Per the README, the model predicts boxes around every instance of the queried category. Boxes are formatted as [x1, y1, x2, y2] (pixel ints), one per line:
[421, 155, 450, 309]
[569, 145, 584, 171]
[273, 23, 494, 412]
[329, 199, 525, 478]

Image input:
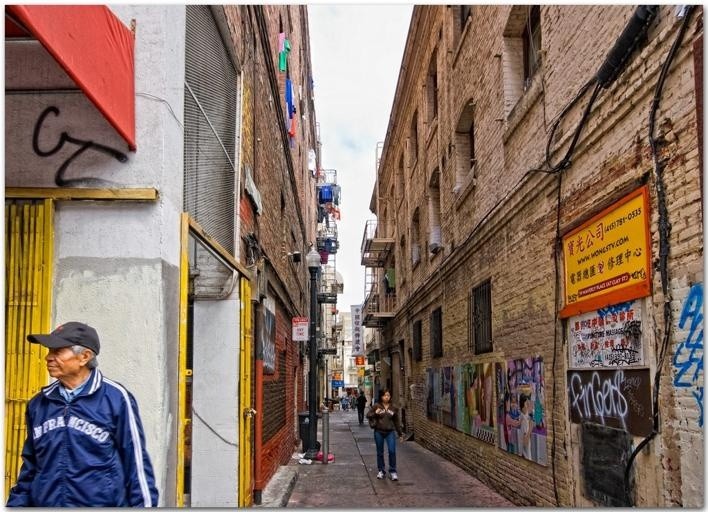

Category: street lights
[305, 245, 322, 461]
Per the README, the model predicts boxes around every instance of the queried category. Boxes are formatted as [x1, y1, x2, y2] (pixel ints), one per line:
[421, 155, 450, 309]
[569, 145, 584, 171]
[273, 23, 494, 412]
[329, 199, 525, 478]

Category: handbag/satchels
[369, 418, 377, 428]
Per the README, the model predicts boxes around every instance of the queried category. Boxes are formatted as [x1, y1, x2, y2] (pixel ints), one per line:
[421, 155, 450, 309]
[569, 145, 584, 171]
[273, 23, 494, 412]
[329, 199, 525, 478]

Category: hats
[27, 321, 100, 355]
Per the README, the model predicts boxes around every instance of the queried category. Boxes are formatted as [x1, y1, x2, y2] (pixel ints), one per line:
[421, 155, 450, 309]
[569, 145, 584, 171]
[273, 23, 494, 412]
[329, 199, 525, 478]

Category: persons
[467, 372, 486, 433]
[366, 388, 403, 480]
[5, 321, 158, 507]
[504, 371, 543, 460]
[356, 390, 368, 424]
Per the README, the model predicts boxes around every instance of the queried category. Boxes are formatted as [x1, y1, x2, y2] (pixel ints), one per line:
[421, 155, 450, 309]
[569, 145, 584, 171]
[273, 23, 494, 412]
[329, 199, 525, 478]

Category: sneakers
[377, 470, 398, 481]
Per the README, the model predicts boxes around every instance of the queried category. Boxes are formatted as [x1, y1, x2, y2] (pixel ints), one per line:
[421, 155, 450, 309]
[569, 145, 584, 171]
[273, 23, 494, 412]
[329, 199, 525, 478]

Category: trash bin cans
[298, 411, 321, 453]
